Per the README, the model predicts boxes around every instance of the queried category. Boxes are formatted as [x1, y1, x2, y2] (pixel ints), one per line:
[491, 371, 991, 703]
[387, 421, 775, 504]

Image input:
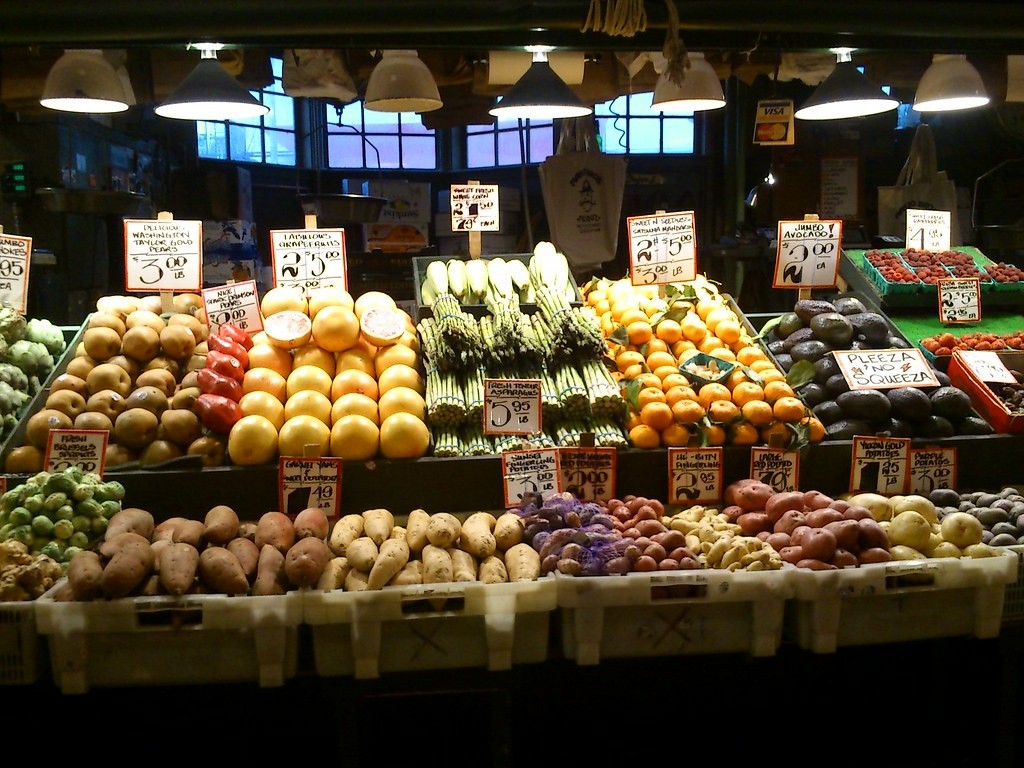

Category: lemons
[228, 286, 430, 460]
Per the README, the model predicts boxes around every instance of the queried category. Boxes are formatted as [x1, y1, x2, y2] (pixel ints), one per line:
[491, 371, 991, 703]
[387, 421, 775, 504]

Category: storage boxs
[947, 267, 994, 306]
[911, 267, 955, 308]
[0, 578, 65, 687]
[742, 312, 996, 494]
[412, 253, 584, 325]
[933, 251, 978, 269]
[948, 349, 1024, 434]
[918, 338, 969, 371]
[304, 513, 556, 679]
[555, 562, 800, 666]
[793, 547, 1018, 653]
[897, 252, 943, 269]
[34, 576, 304, 696]
[861, 251, 906, 282]
[981, 263, 1024, 304]
[874, 268, 922, 309]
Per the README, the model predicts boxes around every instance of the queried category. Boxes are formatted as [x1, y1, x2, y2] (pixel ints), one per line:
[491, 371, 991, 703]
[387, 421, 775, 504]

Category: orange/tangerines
[575, 272, 825, 447]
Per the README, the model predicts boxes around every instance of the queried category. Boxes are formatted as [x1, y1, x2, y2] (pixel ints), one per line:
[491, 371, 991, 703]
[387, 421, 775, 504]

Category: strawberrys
[920, 330, 1024, 356]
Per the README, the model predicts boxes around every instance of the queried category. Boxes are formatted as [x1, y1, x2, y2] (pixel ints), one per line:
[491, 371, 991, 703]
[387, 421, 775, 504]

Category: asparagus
[416, 287, 630, 457]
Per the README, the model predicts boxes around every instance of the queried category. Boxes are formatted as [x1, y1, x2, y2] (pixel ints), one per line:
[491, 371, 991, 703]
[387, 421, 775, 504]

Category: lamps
[364, 49, 443, 113]
[649, 53, 726, 111]
[39, 47, 129, 113]
[795, 47, 901, 121]
[154, 49, 270, 120]
[487, 47, 593, 119]
[913, 53, 989, 111]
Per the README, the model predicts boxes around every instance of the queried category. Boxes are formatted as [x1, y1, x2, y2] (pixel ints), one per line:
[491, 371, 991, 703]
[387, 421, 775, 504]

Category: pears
[6, 293, 253, 466]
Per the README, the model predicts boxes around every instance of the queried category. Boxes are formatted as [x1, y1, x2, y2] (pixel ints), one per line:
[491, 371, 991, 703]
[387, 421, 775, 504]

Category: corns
[422, 242, 575, 308]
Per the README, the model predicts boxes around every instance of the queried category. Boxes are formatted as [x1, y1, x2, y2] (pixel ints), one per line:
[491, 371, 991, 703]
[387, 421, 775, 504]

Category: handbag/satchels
[878, 123, 963, 247]
[538, 115, 626, 274]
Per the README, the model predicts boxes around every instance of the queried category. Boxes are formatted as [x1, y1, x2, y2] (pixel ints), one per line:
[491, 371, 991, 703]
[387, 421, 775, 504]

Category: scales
[971, 159, 1024, 250]
[293, 48, 388, 226]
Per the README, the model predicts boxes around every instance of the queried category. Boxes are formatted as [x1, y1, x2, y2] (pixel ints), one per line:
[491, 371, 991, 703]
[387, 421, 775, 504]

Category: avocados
[764, 297, 994, 442]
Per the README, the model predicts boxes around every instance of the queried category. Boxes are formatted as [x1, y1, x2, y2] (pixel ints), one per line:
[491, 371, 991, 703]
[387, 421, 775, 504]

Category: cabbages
[0, 466, 127, 574]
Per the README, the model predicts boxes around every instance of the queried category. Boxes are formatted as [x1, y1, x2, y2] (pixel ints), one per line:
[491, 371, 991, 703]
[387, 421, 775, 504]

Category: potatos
[67, 508, 541, 634]
[528, 478, 1024, 598]
